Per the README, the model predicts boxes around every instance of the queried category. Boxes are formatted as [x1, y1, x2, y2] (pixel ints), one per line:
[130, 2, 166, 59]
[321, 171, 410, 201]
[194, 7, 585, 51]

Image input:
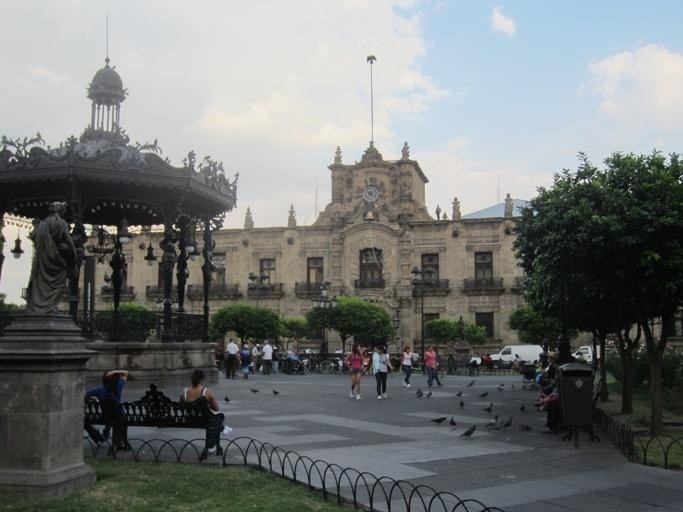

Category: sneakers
[541, 426, 556, 433]
[350, 390, 387, 400]
[207, 445, 217, 453]
[223, 427, 234, 435]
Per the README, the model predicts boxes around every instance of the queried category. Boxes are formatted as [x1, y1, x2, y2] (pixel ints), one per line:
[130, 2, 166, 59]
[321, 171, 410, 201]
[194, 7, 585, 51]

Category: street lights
[248, 270, 269, 308]
[318, 280, 332, 339]
[410, 266, 434, 373]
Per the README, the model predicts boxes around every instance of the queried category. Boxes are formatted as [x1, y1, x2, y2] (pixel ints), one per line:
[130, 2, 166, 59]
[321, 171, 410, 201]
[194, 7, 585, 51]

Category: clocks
[363, 185, 379, 202]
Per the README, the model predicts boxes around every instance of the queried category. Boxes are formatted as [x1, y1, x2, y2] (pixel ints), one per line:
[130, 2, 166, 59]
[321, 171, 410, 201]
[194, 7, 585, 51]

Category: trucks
[571, 346, 603, 363]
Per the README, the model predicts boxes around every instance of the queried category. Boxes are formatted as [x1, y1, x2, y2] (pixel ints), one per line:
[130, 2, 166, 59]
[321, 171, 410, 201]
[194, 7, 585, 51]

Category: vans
[489, 344, 545, 366]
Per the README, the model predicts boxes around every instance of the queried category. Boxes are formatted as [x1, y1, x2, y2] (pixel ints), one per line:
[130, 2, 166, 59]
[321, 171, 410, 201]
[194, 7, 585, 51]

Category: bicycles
[304, 350, 335, 374]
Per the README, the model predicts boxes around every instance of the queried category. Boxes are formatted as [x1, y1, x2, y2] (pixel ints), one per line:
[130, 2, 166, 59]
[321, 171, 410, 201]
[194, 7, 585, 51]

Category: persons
[369, 345, 394, 400]
[343, 342, 363, 400]
[400, 346, 413, 388]
[521, 349, 565, 435]
[224, 338, 522, 383]
[25, 201, 79, 314]
[422, 345, 442, 389]
[178, 370, 233, 454]
[102, 368, 132, 453]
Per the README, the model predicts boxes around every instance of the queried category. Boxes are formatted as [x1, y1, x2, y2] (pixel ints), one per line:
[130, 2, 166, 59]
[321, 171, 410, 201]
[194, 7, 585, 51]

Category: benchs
[85, 382, 225, 461]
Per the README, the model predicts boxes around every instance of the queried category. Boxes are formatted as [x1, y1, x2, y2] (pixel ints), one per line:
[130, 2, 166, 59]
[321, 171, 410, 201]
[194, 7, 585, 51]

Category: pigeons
[416, 383, 531, 438]
[272, 389, 280, 396]
[224, 396, 230, 402]
[250, 388, 259, 394]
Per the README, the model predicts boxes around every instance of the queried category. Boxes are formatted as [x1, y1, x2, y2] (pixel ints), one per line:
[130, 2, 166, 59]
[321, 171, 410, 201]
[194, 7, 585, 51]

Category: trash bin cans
[556, 362, 594, 430]
